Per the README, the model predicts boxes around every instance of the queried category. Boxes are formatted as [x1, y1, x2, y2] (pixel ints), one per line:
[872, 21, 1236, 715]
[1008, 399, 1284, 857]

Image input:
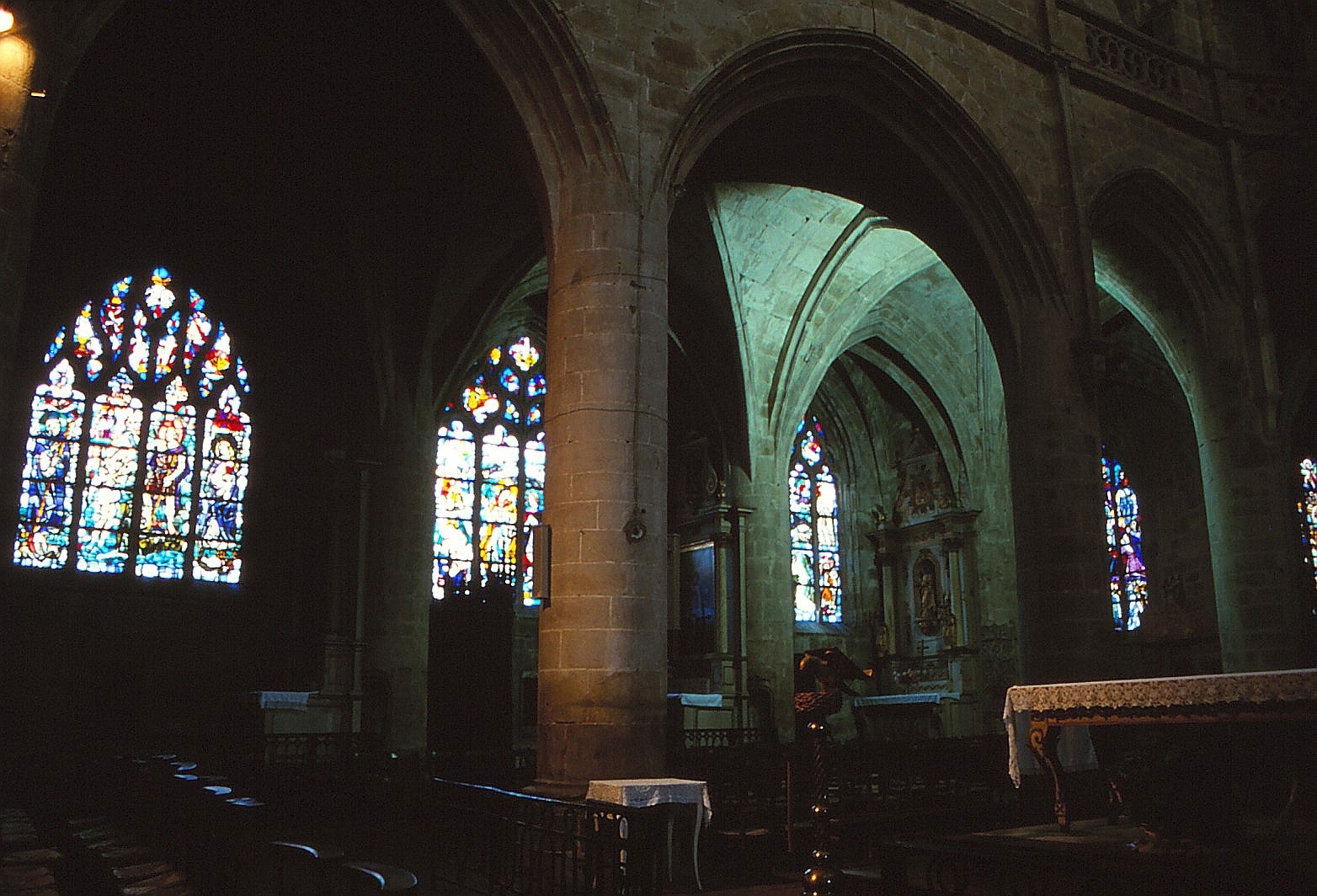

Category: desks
[585, 778, 713, 894]
[847, 692, 961, 740]
[1002, 668, 1316, 829]
